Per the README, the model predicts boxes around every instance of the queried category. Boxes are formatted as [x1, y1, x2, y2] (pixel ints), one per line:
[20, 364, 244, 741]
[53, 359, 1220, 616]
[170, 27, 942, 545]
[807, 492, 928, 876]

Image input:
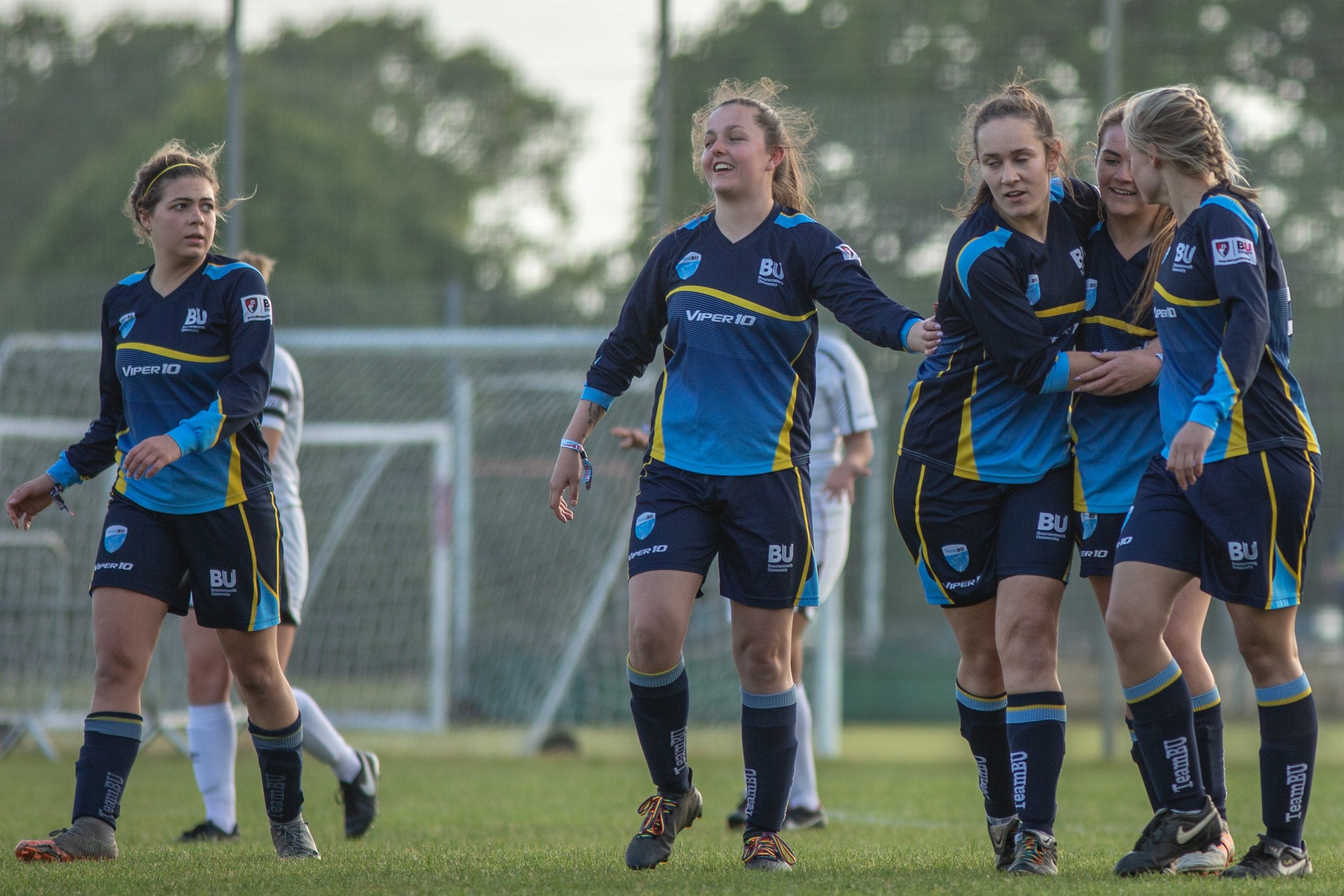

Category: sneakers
[988, 818, 1023, 872]
[1218, 833, 1313, 880]
[1006, 829, 1058, 877]
[1115, 794, 1223, 878]
[625, 784, 703, 870]
[178, 818, 240, 843]
[334, 750, 380, 841]
[15, 827, 118, 865]
[1176, 818, 1236, 876]
[741, 829, 796, 872]
[270, 821, 321, 861]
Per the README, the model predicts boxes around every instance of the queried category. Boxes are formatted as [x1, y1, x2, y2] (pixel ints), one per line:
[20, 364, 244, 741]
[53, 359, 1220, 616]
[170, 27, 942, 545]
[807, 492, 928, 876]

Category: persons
[5, 151, 319, 862]
[179, 249, 379, 843]
[549, 67, 1320, 884]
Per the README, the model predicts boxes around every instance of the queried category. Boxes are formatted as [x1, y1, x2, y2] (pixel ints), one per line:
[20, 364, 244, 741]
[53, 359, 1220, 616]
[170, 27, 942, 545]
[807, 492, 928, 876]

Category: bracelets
[46, 470, 74, 516]
[560, 439, 593, 490]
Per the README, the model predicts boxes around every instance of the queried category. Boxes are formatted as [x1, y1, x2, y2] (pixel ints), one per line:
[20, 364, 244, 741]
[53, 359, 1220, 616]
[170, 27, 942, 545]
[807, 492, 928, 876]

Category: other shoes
[728, 796, 750, 831]
[783, 802, 829, 832]
